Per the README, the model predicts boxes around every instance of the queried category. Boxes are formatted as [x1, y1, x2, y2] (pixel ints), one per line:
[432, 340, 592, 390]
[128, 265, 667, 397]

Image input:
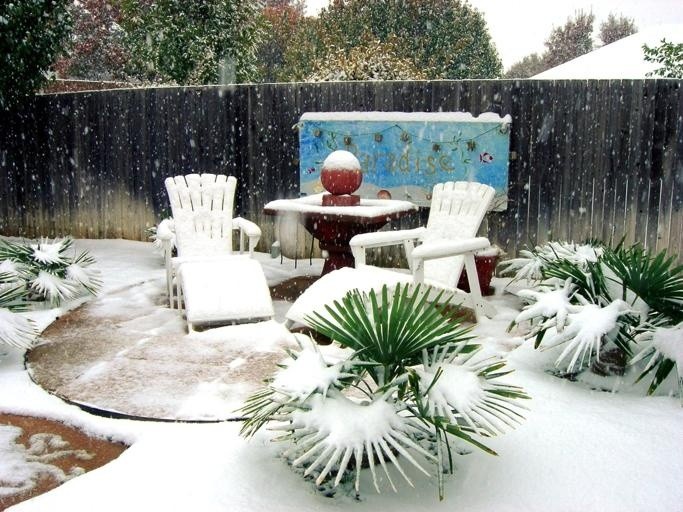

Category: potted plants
[495, 231, 683, 400]
